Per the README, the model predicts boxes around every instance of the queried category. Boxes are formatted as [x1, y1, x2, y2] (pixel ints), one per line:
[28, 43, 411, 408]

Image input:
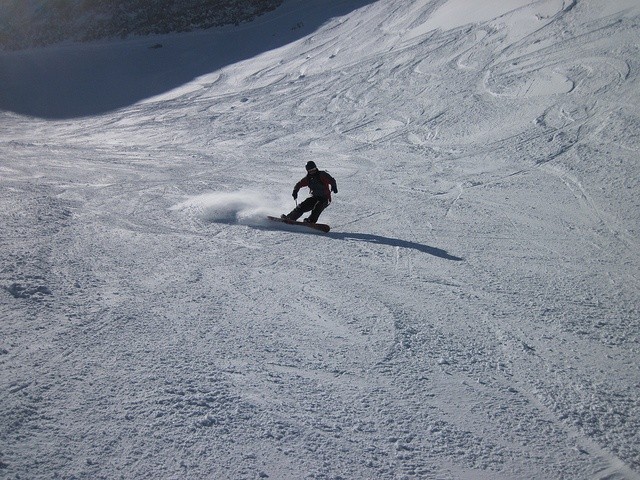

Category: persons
[281, 160, 338, 224]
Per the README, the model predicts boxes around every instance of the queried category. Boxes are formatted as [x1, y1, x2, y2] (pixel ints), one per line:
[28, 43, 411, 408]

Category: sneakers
[304, 218, 315, 224]
[281, 214, 296, 223]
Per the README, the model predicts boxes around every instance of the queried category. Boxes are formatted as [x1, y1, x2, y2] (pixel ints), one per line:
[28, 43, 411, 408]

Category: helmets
[305, 161, 317, 173]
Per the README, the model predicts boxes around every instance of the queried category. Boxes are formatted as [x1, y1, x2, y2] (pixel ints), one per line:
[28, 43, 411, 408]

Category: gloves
[330, 187, 338, 194]
[292, 191, 298, 200]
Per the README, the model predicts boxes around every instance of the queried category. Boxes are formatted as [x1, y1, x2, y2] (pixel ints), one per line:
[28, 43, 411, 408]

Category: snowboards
[267, 216, 330, 233]
